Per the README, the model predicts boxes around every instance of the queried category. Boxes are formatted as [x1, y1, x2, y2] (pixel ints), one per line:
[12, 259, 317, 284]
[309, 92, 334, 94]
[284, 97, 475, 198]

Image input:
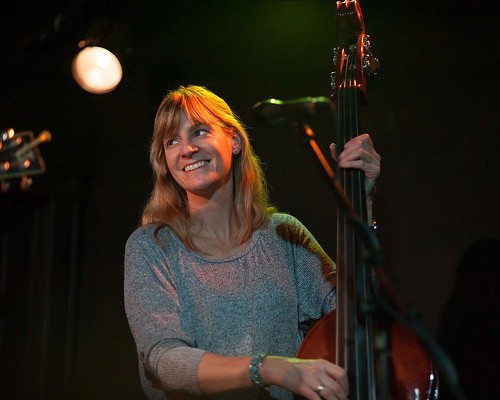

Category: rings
[317, 385, 325, 393]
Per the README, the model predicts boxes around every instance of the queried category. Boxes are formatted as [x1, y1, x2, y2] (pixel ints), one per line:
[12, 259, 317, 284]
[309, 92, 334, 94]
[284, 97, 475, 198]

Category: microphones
[252, 97, 337, 129]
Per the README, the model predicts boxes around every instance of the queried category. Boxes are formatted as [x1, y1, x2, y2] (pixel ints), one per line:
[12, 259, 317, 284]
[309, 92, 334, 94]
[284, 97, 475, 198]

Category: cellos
[284, 1, 443, 400]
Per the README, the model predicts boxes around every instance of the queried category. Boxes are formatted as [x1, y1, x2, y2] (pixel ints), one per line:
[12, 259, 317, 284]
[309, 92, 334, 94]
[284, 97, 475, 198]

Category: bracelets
[249, 353, 269, 389]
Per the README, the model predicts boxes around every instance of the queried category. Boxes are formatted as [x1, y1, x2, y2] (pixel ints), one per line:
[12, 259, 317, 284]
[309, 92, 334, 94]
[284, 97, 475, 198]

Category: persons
[122, 84, 380, 400]
